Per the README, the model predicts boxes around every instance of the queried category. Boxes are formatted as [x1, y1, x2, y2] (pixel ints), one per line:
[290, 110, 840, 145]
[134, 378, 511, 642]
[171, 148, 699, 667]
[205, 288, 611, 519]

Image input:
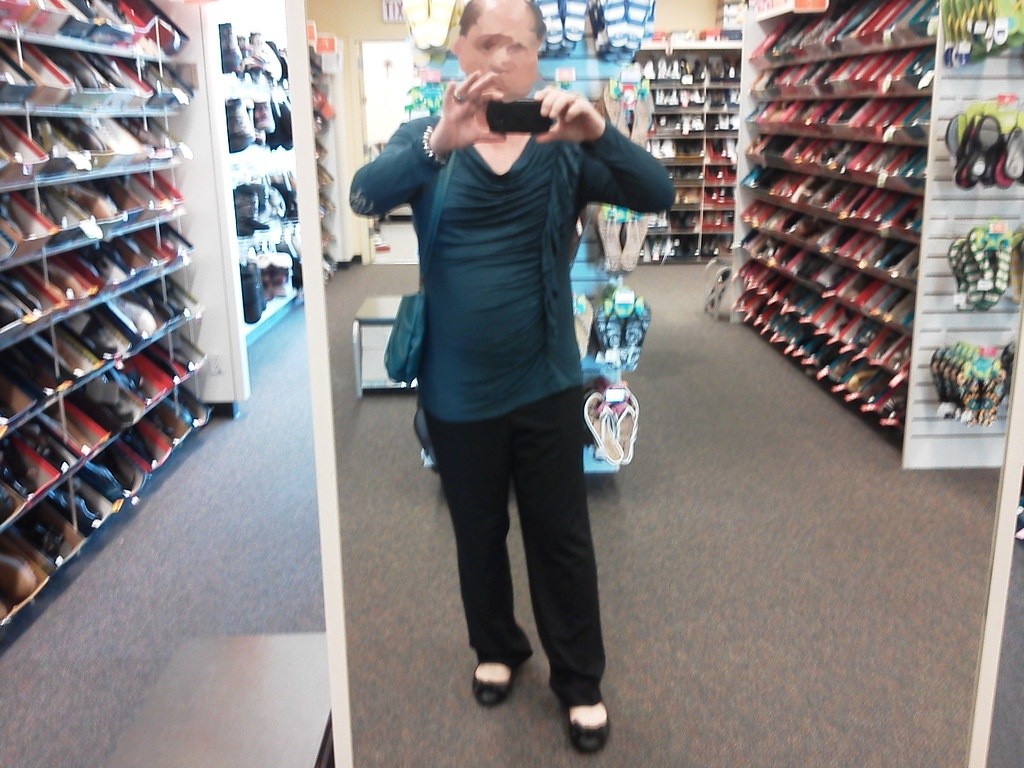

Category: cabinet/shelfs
[306, 0, 1024, 768]
[644, 0, 1022, 470]
[0, 0, 300, 654]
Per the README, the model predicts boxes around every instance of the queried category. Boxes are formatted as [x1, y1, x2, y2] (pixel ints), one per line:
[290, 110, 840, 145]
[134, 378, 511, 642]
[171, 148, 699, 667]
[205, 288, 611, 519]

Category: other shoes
[567, 702, 611, 754]
[472, 662, 518, 708]
[217, 23, 303, 324]
[620, 31, 741, 263]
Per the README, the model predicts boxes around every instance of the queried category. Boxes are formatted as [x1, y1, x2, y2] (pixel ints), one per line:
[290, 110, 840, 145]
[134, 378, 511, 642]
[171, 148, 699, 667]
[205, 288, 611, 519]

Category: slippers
[572, 284, 652, 372]
[945, 100, 1024, 191]
[930, 340, 1016, 429]
[597, 203, 649, 273]
[600, 77, 655, 151]
[582, 383, 640, 466]
[948, 220, 1024, 313]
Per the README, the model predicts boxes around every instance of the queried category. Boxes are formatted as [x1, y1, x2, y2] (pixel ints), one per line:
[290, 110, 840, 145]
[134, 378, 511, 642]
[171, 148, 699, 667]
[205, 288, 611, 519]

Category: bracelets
[424, 125, 449, 165]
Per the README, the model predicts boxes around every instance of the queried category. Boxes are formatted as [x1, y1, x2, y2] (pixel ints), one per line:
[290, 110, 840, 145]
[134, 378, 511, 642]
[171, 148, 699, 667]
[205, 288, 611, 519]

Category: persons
[348, 0, 676, 753]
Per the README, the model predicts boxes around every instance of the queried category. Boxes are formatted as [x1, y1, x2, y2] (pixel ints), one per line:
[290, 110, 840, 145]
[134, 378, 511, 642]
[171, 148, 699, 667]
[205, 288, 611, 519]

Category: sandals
[534, 0, 656, 65]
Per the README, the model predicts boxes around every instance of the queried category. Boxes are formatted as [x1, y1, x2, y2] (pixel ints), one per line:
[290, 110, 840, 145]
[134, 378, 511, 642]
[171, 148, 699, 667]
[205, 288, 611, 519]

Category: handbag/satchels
[383, 291, 425, 389]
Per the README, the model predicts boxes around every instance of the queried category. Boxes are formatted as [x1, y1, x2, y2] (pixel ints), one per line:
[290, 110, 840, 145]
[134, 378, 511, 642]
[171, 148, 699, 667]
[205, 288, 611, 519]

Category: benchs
[352, 295, 419, 398]
[104, 632, 336, 768]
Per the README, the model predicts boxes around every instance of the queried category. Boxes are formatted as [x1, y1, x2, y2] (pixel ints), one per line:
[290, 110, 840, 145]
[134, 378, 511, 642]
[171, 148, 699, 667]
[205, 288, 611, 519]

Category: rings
[452, 92, 466, 103]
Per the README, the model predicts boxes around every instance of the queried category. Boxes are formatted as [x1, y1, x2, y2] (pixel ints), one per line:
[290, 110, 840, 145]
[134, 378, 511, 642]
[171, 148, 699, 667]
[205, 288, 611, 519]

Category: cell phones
[488, 98, 555, 133]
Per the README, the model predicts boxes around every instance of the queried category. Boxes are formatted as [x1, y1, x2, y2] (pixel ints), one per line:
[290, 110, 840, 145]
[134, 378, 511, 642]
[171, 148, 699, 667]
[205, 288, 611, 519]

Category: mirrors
[284, 0, 1024, 768]
